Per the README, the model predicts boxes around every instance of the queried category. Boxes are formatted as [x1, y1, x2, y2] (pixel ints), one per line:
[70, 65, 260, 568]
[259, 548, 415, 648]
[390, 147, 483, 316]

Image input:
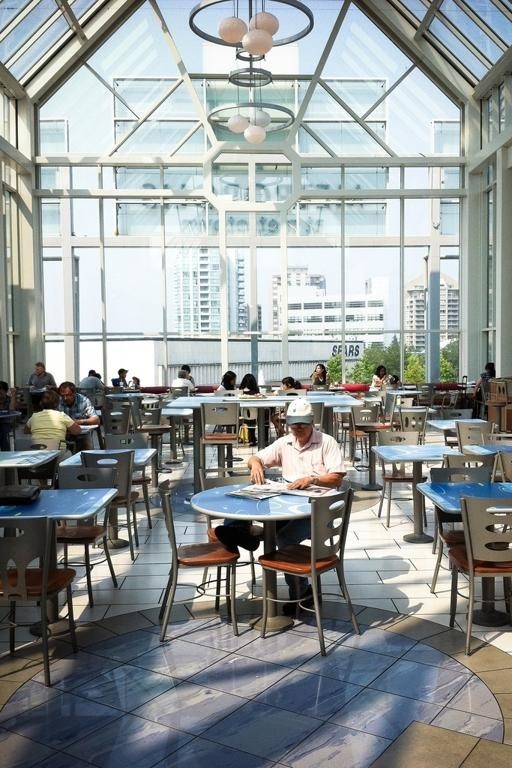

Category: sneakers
[249, 440, 257, 446]
[215, 524, 260, 551]
[282, 585, 314, 613]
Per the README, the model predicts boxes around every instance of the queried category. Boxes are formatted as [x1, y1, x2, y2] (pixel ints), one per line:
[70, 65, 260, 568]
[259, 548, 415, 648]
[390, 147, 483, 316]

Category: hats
[286, 398, 313, 424]
[118, 368, 128, 374]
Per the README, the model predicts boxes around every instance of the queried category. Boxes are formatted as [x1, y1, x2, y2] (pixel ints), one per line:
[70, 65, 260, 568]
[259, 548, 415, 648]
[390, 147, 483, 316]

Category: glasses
[290, 423, 307, 429]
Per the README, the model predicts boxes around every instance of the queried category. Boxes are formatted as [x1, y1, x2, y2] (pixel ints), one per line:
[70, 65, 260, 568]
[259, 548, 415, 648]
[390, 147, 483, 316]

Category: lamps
[207, 46, 295, 143]
[188, 0, 315, 55]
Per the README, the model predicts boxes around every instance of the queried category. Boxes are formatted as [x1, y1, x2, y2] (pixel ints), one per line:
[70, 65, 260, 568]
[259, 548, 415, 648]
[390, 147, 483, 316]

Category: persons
[389, 375, 402, 387]
[471, 362, 496, 420]
[311, 363, 327, 385]
[130, 375, 140, 390]
[171, 370, 194, 396]
[238, 373, 259, 446]
[271, 377, 302, 439]
[215, 371, 237, 396]
[93, 373, 108, 387]
[54, 382, 101, 451]
[214, 398, 345, 617]
[79, 368, 111, 397]
[26, 360, 59, 411]
[117, 367, 129, 388]
[371, 365, 395, 394]
[181, 365, 194, 387]
[0, 380, 18, 452]
[24, 390, 82, 489]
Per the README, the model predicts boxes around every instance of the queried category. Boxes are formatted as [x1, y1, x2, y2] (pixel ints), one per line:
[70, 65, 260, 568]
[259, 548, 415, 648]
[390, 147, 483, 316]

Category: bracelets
[83, 419, 87, 424]
[11, 396, 16, 400]
[311, 474, 320, 486]
